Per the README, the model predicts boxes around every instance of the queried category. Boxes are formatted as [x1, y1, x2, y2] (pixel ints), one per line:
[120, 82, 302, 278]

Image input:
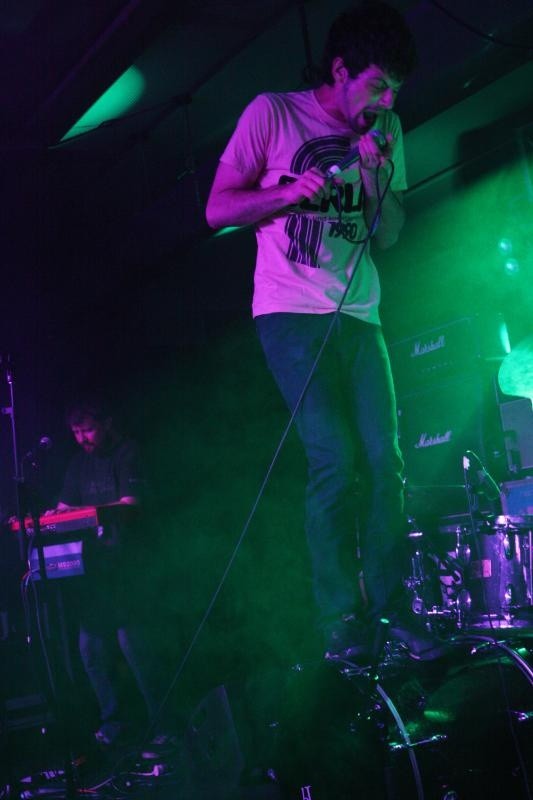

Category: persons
[205, 32, 444, 665]
[42, 402, 170, 791]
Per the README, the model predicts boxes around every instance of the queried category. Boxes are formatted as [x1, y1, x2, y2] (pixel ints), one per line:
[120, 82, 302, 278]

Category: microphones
[467, 450, 501, 501]
[325, 130, 388, 179]
[25, 437, 51, 460]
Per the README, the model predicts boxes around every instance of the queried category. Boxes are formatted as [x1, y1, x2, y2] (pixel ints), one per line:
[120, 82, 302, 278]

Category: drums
[459, 513, 532, 629]
[258, 635, 533, 799]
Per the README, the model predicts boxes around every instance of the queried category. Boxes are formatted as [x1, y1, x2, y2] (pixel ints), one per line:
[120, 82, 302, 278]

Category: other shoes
[94, 716, 122, 743]
[324, 623, 382, 660]
[371, 615, 438, 661]
[140, 729, 177, 759]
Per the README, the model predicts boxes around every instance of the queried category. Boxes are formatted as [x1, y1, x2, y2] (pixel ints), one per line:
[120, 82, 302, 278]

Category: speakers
[392, 318, 533, 518]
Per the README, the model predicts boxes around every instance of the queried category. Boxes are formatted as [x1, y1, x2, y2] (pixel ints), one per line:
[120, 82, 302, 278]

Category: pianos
[10, 501, 134, 717]
